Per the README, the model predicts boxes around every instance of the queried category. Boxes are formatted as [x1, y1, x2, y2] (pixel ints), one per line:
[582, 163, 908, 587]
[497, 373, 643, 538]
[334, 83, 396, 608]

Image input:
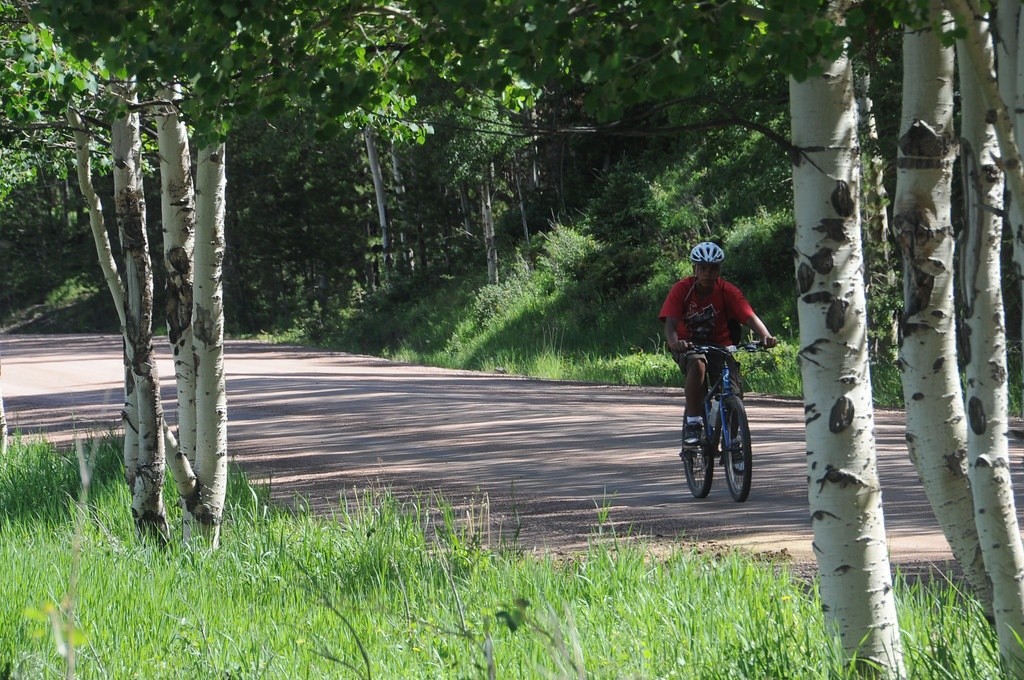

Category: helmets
[691, 241, 725, 265]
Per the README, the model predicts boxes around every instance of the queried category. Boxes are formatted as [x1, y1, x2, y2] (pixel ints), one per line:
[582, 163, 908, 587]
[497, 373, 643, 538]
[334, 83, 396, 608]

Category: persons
[657, 242, 780, 473]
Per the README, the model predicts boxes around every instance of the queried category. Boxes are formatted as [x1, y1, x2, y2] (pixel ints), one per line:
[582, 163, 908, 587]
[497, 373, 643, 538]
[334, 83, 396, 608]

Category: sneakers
[727, 435, 745, 475]
[683, 420, 705, 452]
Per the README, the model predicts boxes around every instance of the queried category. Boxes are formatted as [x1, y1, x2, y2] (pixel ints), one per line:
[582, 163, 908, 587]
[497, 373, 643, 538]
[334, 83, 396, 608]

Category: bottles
[709, 395, 721, 427]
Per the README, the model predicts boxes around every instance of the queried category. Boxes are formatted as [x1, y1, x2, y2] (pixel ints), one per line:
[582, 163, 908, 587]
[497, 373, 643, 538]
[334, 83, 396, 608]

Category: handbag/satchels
[729, 319, 742, 346]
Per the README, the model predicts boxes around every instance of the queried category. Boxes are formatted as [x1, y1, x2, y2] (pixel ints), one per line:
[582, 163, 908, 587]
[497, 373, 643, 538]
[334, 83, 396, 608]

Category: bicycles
[675, 342, 771, 503]
